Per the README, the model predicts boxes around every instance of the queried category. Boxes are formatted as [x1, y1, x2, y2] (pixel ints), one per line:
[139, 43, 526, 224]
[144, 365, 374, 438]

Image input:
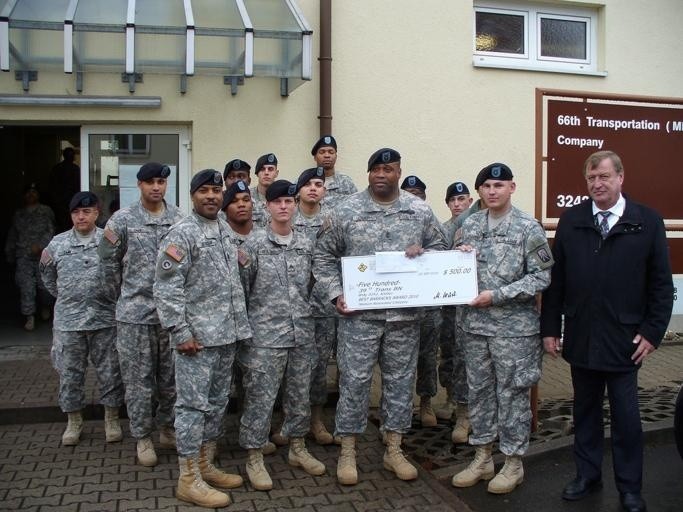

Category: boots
[137, 423, 178, 467]
[25, 309, 50, 330]
[420, 397, 437, 427]
[452, 443, 495, 488]
[104, 404, 123, 442]
[337, 435, 357, 485]
[452, 404, 472, 442]
[198, 444, 243, 488]
[436, 387, 456, 419]
[62, 410, 84, 445]
[383, 432, 418, 480]
[245, 405, 333, 490]
[176, 454, 230, 507]
[488, 455, 524, 493]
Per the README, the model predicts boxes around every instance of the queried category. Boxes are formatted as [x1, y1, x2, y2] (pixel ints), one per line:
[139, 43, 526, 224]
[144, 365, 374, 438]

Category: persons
[3, 180, 64, 332]
[47, 148, 80, 232]
[539, 150, 673, 512]
[96, 162, 189, 466]
[451, 163, 554, 495]
[152, 168, 244, 509]
[38, 193, 123, 446]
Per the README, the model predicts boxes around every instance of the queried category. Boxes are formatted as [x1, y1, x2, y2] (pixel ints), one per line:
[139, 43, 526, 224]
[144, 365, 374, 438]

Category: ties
[599, 213, 613, 239]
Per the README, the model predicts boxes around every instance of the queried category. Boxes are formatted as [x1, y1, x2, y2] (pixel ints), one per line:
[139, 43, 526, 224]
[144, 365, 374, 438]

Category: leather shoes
[620, 492, 646, 512]
[563, 477, 603, 499]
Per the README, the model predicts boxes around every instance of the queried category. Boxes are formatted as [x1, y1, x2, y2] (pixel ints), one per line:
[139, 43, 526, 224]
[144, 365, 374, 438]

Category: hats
[368, 148, 400, 172]
[297, 167, 325, 190]
[265, 180, 296, 201]
[224, 159, 250, 180]
[401, 176, 426, 190]
[475, 163, 512, 190]
[70, 191, 98, 211]
[221, 181, 250, 211]
[190, 169, 223, 193]
[446, 182, 470, 204]
[312, 136, 337, 156]
[137, 162, 170, 180]
[255, 153, 277, 174]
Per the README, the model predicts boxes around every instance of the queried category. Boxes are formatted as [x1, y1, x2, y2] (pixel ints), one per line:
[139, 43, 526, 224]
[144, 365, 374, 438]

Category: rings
[180, 351, 188, 358]
